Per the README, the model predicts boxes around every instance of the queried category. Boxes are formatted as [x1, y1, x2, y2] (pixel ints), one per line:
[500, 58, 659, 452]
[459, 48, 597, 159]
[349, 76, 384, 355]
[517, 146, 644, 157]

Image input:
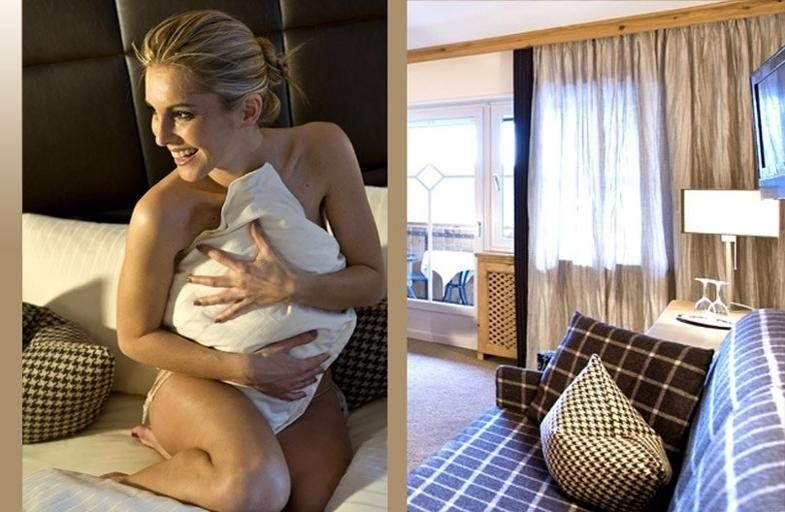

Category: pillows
[22, 302, 116, 443]
[539, 353, 674, 512]
[525, 309, 715, 456]
[327, 300, 388, 414]
[163, 161, 358, 437]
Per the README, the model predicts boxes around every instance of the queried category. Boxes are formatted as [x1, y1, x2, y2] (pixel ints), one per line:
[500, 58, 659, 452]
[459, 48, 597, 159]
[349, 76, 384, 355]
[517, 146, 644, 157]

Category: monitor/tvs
[748, 43, 785, 199]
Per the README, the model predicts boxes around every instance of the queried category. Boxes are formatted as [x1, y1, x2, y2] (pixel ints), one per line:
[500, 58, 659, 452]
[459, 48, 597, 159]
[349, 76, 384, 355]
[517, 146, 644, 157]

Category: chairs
[407, 231, 428, 300]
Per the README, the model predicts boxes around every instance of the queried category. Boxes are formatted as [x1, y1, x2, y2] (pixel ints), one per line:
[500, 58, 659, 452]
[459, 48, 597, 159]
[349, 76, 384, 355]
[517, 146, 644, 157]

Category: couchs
[407, 309, 785, 512]
[22, 214, 389, 512]
[324, 184, 387, 278]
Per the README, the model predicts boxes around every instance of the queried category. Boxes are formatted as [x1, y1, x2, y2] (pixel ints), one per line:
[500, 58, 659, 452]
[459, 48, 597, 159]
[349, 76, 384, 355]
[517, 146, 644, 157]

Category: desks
[644, 299, 751, 351]
[421, 249, 475, 304]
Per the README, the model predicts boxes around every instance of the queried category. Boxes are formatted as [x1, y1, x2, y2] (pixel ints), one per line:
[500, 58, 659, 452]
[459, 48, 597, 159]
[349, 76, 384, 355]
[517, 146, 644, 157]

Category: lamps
[681, 188, 780, 312]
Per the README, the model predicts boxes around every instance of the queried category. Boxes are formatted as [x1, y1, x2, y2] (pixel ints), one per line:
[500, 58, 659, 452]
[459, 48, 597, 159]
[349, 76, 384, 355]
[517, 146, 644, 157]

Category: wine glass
[693, 275, 731, 327]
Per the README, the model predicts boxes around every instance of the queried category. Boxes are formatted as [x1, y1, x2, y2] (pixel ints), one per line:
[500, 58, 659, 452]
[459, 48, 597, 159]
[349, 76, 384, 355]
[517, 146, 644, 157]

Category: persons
[97, 7, 387, 512]
